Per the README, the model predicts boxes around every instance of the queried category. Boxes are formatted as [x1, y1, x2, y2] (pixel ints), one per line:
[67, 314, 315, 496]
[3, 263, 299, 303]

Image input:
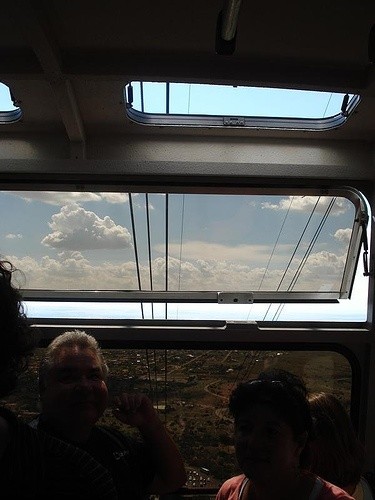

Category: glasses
[244, 379, 288, 391]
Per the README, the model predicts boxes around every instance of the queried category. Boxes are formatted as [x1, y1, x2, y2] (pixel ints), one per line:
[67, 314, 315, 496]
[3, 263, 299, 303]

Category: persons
[215, 367, 357, 500]
[0, 261, 120, 500]
[306, 392, 375, 500]
[37, 329, 187, 500]
